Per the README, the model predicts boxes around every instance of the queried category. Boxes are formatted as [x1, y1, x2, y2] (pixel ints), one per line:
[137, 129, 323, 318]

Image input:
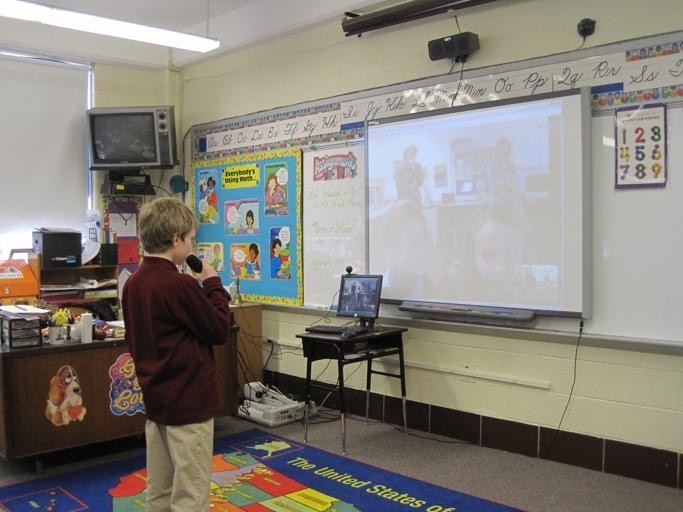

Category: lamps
[0, 0, 222, 70]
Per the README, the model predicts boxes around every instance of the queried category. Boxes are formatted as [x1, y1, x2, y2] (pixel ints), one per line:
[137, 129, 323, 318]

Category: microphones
[186, 254, 231, 303]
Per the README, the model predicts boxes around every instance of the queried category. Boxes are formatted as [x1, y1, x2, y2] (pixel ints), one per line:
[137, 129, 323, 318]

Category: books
[41, 276, 117, 300]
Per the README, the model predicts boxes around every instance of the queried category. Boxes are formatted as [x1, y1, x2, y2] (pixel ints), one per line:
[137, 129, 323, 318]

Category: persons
[210, 244, 222, 271]
[270, 238, 282, 278]
[120, 194, 235, 512]
[204, 176, 218, 209]
[244, 243, 259, 275]
[198, 248, 204, 258]
[372, 136, 564, 303]
[341, 278, 376, 313]
[264, 172, 285, 204]
[243, 208, 254, 229]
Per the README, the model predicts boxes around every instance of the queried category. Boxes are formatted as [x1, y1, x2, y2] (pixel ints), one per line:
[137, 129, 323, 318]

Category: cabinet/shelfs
[1, 325, 241, 474]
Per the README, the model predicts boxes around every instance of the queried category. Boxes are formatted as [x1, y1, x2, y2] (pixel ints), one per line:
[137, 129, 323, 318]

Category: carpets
[0, 427, 526, 512]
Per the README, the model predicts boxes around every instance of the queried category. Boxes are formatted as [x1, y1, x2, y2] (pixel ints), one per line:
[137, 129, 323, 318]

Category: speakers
[426, 31, 478, 61]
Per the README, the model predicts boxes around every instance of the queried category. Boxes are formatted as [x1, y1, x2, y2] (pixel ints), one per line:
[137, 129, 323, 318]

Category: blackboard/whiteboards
[191, 31, 683, 355]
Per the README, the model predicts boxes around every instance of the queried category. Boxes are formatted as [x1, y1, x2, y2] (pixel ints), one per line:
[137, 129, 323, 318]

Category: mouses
[340, 331, 355, 338]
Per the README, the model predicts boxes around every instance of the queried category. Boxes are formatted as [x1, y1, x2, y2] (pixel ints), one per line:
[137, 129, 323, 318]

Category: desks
[294, 328, 411, 456]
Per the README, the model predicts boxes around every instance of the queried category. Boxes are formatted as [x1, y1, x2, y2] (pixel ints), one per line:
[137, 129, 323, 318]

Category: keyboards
[305, 325, 366, 335]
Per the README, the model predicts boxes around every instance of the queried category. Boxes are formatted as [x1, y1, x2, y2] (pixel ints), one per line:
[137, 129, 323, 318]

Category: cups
[41, 327, 65, 345]
[81, 313, 92, 343]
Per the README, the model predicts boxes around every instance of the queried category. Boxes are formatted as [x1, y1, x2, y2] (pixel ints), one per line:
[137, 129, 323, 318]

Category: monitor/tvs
[85, 104, 175, 172]
[337, 274, 383, 327]
